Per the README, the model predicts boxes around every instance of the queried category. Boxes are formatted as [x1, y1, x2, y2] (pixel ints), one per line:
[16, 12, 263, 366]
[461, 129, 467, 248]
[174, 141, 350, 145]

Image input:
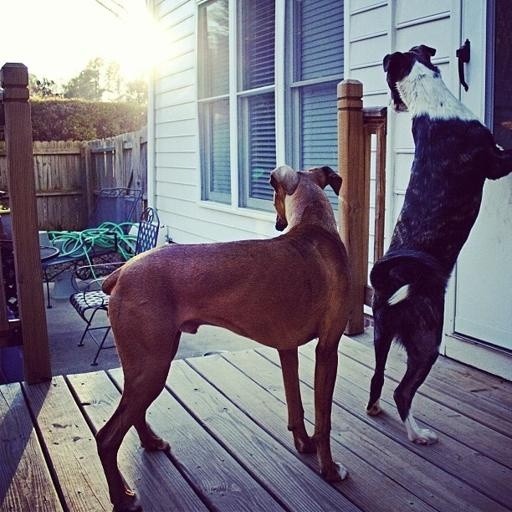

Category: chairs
[68, 205, 160, 368]
[37, 245, 61, 309]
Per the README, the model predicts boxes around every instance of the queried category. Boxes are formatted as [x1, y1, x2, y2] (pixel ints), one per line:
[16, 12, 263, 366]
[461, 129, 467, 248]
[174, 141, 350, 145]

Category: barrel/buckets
[51, 269, 75, 301]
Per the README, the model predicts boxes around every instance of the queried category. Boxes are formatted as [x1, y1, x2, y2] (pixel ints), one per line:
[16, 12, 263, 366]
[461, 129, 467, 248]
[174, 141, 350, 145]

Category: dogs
[364, 44, 512, 446]
[95, 164, 354, 512]
